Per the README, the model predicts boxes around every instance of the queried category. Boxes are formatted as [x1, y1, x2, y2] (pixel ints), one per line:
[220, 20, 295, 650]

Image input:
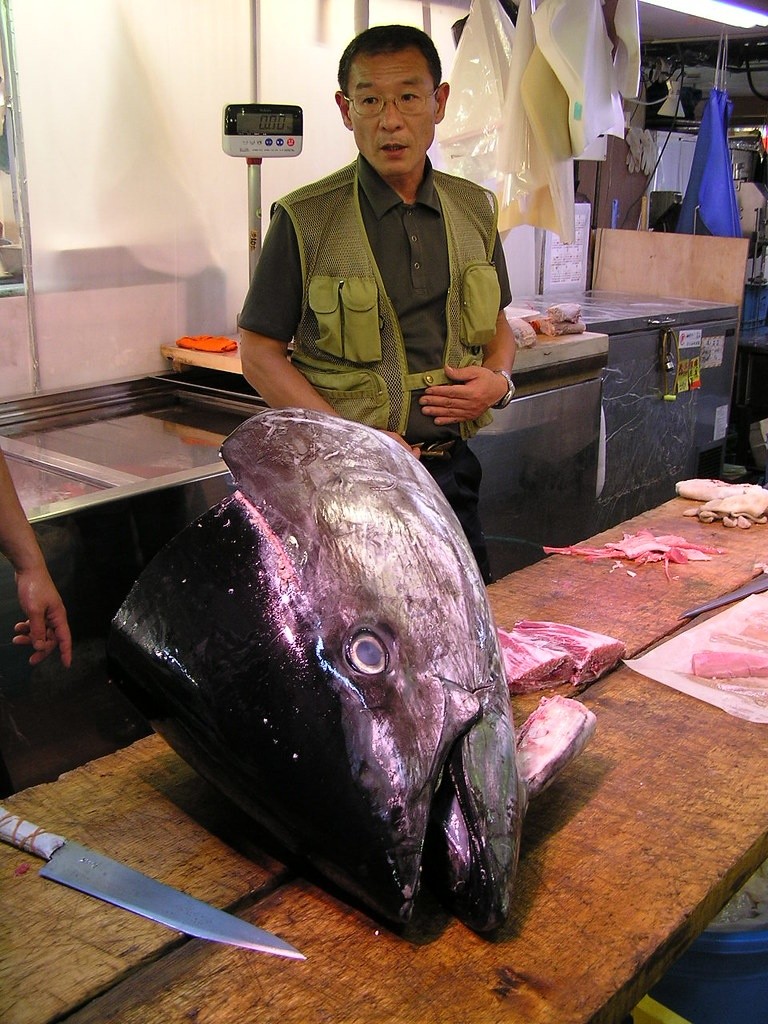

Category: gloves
[625, 127, 660, 176]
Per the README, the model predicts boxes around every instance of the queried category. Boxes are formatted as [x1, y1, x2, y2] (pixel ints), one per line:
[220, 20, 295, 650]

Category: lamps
[657, 81, 685, 118]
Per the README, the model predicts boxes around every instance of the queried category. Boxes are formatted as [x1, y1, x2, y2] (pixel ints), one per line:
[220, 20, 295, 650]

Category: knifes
[0, 806, 305, 960]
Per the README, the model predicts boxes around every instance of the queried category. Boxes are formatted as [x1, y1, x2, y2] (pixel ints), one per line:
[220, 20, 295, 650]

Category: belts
[408, 439, 455, 460]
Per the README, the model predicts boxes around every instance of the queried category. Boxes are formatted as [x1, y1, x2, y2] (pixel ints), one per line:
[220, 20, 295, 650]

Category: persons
[238, 25, 517, 587]
[0, 447, 72, 670]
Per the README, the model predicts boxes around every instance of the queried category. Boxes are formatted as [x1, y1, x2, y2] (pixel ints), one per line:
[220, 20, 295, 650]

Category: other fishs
[104, 407, 523, 936]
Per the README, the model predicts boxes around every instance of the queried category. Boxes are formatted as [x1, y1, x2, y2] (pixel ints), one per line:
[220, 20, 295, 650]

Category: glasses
[342, 86, 441, 117]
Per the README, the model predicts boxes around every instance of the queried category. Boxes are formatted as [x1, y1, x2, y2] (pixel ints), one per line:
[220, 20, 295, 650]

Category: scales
[159, 103, 304, 376]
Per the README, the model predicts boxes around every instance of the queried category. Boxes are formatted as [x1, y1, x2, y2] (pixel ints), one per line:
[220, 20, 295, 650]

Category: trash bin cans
[629, 858, 768, 1024]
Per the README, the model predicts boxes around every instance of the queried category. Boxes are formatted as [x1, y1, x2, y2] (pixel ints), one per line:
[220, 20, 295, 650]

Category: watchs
[492, 367, 516, 409]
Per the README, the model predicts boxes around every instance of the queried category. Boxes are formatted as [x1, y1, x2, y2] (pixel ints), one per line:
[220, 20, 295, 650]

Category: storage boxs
[740, 285, 768, 330]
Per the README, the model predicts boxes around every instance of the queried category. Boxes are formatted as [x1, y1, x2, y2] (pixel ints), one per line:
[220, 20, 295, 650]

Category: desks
[0, 494, 767, 1023]
